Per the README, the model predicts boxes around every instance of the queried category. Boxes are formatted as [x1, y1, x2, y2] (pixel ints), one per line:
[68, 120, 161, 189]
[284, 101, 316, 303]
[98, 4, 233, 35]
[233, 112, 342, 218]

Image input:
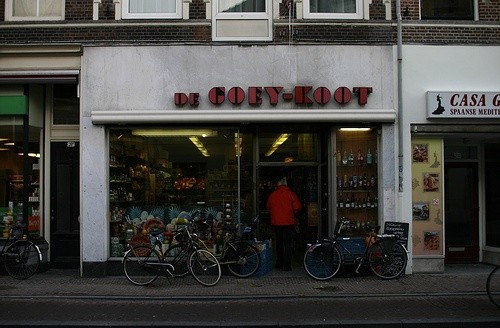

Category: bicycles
[303, 216, 410, 281]
[161, 211, 262, 279]
[485, 265, 500, 309]
[0, 226, 42, 281]
[121, 219, 222, 287]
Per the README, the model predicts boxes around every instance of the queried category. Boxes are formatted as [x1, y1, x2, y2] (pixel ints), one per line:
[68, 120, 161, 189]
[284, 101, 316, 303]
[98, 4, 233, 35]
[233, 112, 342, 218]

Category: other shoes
[275, 265, 292, 271]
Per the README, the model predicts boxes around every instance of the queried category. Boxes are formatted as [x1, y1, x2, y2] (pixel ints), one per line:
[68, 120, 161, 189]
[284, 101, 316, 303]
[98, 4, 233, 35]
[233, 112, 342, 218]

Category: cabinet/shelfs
[110, 154, 146, 237]
[206, 179, 238, 205]
[337, 163, 378, 210]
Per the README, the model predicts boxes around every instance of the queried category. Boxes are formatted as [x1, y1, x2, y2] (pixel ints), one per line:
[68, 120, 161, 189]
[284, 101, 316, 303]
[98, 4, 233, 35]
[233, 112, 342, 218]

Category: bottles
[118, 186, 127, 200]
[338, 216, 376, 229]
[338, 173, 376, 187]
[374, 149, 377, 163]
[336, 194, 377, 207]
[337, 148, 373, 164]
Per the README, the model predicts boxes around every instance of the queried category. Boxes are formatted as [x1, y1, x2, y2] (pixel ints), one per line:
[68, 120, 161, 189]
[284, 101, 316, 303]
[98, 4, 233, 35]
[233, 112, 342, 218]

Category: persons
[266, 176, 302, 272]
